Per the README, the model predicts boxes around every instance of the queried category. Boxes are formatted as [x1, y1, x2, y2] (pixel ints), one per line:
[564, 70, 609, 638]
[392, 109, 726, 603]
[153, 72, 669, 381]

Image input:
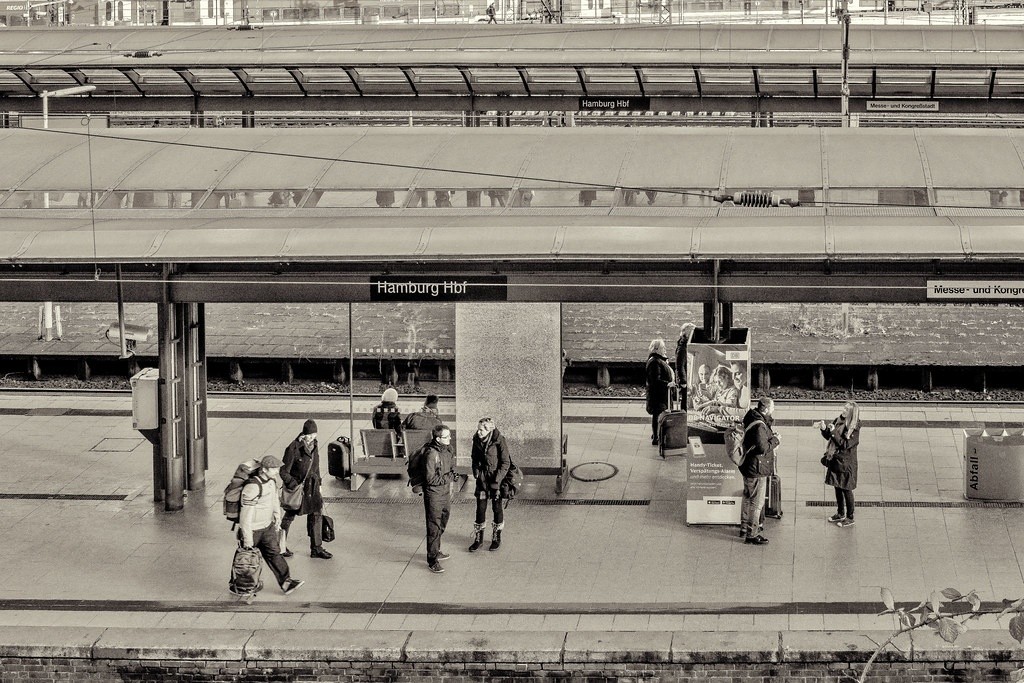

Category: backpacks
[723, 420, 767, 466]
[404, 442, 440, 496]
[221, 458, 277, 531]
[228, 546, 264, 597]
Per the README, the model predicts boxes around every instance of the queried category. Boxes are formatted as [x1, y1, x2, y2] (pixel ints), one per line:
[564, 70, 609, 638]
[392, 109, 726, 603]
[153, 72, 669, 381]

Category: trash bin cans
[963, 427, 1024, 502]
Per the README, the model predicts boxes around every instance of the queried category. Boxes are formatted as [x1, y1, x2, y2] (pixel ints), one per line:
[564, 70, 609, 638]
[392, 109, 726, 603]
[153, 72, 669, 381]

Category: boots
[488, 520, 505, 551]
[469, 521, 486, 552]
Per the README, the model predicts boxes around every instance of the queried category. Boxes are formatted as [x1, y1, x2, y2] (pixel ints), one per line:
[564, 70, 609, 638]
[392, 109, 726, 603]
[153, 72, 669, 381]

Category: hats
[425, 394, 437, 405]
[260, 454, 285, 469]
[380, 387, 399, 404]
[303, 419, 318, 435]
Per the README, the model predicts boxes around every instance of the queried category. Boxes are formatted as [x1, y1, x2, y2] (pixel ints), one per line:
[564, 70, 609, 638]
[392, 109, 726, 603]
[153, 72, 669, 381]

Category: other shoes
[428, 560, 445, 573]
[437, 551, 451, 560]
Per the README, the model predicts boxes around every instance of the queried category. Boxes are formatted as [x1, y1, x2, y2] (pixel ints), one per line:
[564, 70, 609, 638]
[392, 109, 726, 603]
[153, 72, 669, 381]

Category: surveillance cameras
[108, 322, 153, 341]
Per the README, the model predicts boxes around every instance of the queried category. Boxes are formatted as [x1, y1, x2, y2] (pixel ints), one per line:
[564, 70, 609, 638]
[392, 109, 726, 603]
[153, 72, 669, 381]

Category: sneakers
[828, 513, 846, 522]
[281, 577, 305, 596]
[836, 518, 856, 528]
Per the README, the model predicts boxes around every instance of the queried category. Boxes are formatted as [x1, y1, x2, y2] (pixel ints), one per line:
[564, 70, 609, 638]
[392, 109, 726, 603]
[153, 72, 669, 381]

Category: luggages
[328, 435, 353, 481]
[656, 383, 689, 460]
[765, 452, 783, 519]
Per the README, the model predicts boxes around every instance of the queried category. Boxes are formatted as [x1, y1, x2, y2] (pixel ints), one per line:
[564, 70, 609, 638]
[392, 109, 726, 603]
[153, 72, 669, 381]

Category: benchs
[351, 428, 435, 476]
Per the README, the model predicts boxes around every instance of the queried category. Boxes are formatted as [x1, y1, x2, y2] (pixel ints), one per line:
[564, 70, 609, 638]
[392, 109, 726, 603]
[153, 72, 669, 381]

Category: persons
[693, 361, 748, 421]
[737, 397, 781, 545]
[228, 456, 305, 594]
[488, 2, 498, 24]
[468, 418, 510, 551]
[243, 5, 249, 26]
[815, 402, 862, 527]
[645, 340, 675, 445]
[401, 394, 443, 431]
[422, 425, 459, 573]
[372, 388, 403, 453]
[674, 323, 695, 411]
[279, 419, 333, 560]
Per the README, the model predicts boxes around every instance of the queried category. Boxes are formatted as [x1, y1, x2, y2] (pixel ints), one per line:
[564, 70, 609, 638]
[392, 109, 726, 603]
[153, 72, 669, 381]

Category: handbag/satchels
[278, 480, 304, 511]
[499, 462, 524, 510]
[306, 502, 335, 542]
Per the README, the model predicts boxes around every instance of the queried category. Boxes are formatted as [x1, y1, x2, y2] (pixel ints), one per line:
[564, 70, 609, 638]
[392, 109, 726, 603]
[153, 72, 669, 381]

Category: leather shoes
[744, 535, 769, 546]
[310, 549, 333, 559]
[283, 547, 294, 558]
[739, 529, 763, 538]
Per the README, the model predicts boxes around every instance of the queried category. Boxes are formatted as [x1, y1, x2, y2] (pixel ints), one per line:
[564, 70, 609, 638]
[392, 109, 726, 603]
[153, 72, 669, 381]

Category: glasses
[439, 433, 452, 439]
